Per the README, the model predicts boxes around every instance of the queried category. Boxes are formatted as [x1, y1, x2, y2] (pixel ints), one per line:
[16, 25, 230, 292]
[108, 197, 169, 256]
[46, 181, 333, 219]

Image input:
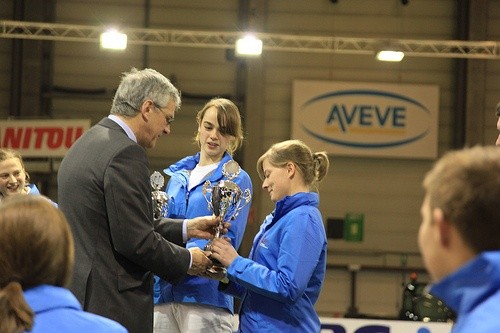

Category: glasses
[153, 102, 176, 125]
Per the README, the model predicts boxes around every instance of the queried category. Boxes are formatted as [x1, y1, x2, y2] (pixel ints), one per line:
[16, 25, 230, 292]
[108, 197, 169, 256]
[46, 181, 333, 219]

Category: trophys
[198, 160, 253, 284]
[150, 171, 170, 232]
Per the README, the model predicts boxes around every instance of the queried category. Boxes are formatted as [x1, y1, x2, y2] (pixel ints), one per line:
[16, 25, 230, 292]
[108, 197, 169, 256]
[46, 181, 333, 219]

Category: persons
[0, 67, 330, 333]
[416, 145, 500, 332]
